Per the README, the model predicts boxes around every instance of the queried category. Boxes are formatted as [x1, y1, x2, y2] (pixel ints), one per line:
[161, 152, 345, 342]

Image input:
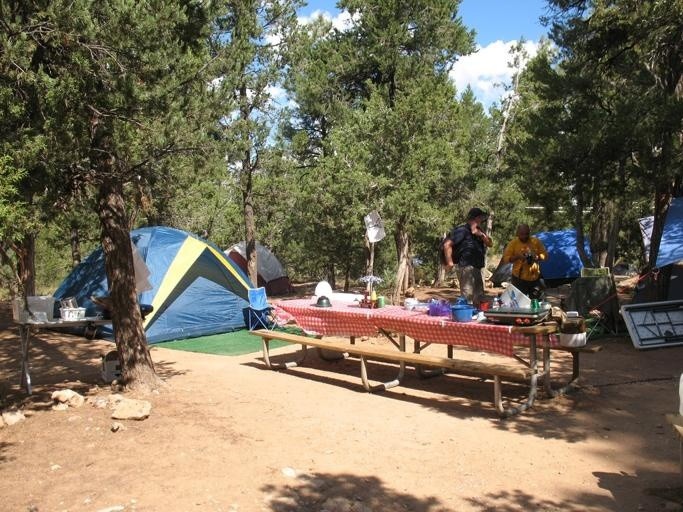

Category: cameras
[525, 251, 534, 266]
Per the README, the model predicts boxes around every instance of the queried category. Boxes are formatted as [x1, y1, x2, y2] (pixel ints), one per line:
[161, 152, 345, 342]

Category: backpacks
[433, 225, 467, 264]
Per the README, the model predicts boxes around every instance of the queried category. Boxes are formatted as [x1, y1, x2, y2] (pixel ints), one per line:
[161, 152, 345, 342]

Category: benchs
[520, 342, 601, 386]
[249, 328, 535, 418]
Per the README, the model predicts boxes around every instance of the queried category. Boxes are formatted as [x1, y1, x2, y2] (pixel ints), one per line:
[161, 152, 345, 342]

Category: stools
[585, 314, 609, 341]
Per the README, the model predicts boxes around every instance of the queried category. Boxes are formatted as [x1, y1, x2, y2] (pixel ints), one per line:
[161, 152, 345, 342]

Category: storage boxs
[26, 296, 54, 320]
[101, 353, 122, 383]
[59, 307, 87, 319]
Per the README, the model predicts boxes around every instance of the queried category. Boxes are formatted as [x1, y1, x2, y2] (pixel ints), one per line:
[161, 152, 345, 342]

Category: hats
[469, 208, 486, 216]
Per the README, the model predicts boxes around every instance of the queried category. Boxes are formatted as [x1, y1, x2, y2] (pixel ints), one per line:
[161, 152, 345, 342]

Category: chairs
[247, 287, 286, 331]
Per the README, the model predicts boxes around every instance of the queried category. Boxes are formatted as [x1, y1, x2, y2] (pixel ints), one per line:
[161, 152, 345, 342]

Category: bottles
[530, 294, 538, 308]
[492, 298, 499, 308]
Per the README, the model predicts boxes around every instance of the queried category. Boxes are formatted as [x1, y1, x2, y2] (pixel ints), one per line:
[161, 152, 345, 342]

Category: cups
[541, 302, 551, 309]
[370, 291, 377, 300]
[378, 296, 385, 308]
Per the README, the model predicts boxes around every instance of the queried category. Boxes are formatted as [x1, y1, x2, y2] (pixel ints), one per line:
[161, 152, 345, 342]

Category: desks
[270, 299, 560, 405]
[571, 275, 618, 333]
[13, 317, 146, 395]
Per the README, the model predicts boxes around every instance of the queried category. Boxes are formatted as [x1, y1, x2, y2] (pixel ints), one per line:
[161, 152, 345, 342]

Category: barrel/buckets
[60, 296, 78, 308]
[452, 305, 480, 321]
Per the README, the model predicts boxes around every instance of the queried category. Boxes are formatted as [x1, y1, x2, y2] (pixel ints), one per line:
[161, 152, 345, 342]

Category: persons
[502, 224, 549, 303]
[441, 207, 492, 307]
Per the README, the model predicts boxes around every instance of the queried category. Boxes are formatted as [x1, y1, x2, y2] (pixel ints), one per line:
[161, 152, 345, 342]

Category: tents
[488, 230, 591, 292]
[50, 225, 269, 346]
[221, 240, 294, 297]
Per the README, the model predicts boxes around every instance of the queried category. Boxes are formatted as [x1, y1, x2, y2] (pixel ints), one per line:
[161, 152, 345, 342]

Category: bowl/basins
[450, 305, 474, 322]
